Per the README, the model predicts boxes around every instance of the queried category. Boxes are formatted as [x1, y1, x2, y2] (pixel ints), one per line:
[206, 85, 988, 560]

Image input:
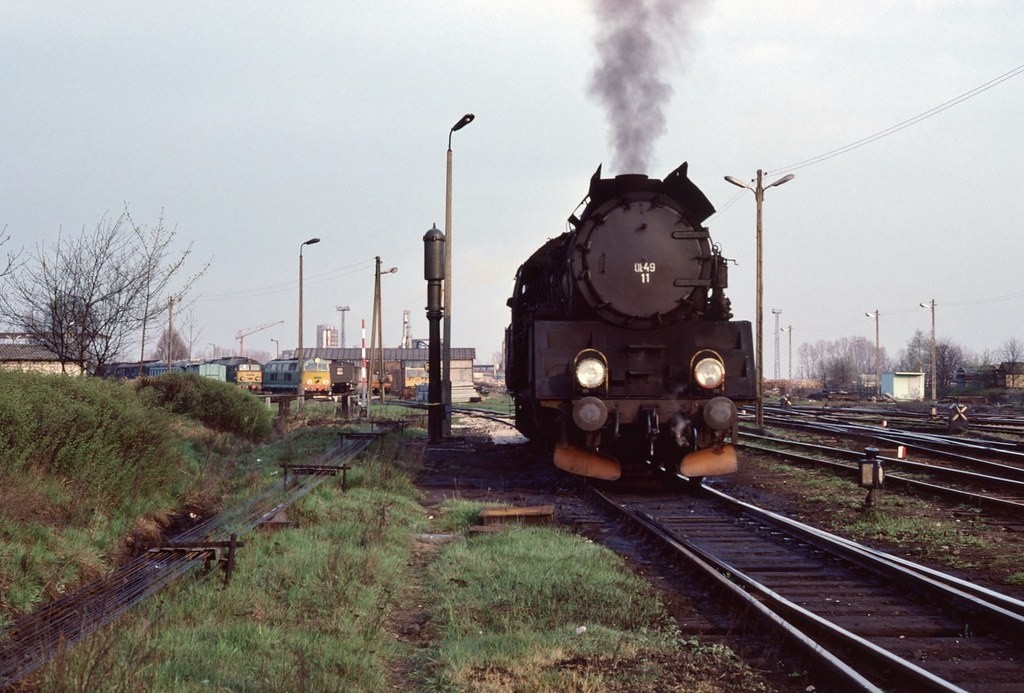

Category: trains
[507, 158, 760, 485]
[92, 354, 356, 401]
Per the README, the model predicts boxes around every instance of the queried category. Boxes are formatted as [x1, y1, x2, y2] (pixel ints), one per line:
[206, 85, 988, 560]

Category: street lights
[724, 171, 795, 426]
[865, 310, 880, 400]
[780, 324, 794, 404]
[297, 237, 320, 407]
[920, 300, 938, 401]
[375, 268, 399, 404]
